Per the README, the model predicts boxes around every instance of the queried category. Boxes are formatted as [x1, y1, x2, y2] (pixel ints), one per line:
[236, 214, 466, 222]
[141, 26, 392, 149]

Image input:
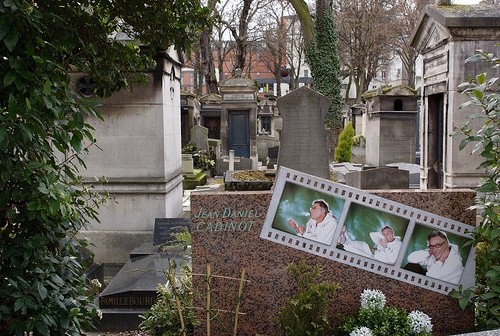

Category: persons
[402, 229, 465, 286]
[338, 225, 403, 266]
[287, 199, 338, 247]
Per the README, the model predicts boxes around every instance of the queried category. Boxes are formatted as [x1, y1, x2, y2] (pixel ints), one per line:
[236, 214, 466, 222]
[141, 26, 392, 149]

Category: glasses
[309, 205, 322, 210]
[428, 240, 445, 249]
